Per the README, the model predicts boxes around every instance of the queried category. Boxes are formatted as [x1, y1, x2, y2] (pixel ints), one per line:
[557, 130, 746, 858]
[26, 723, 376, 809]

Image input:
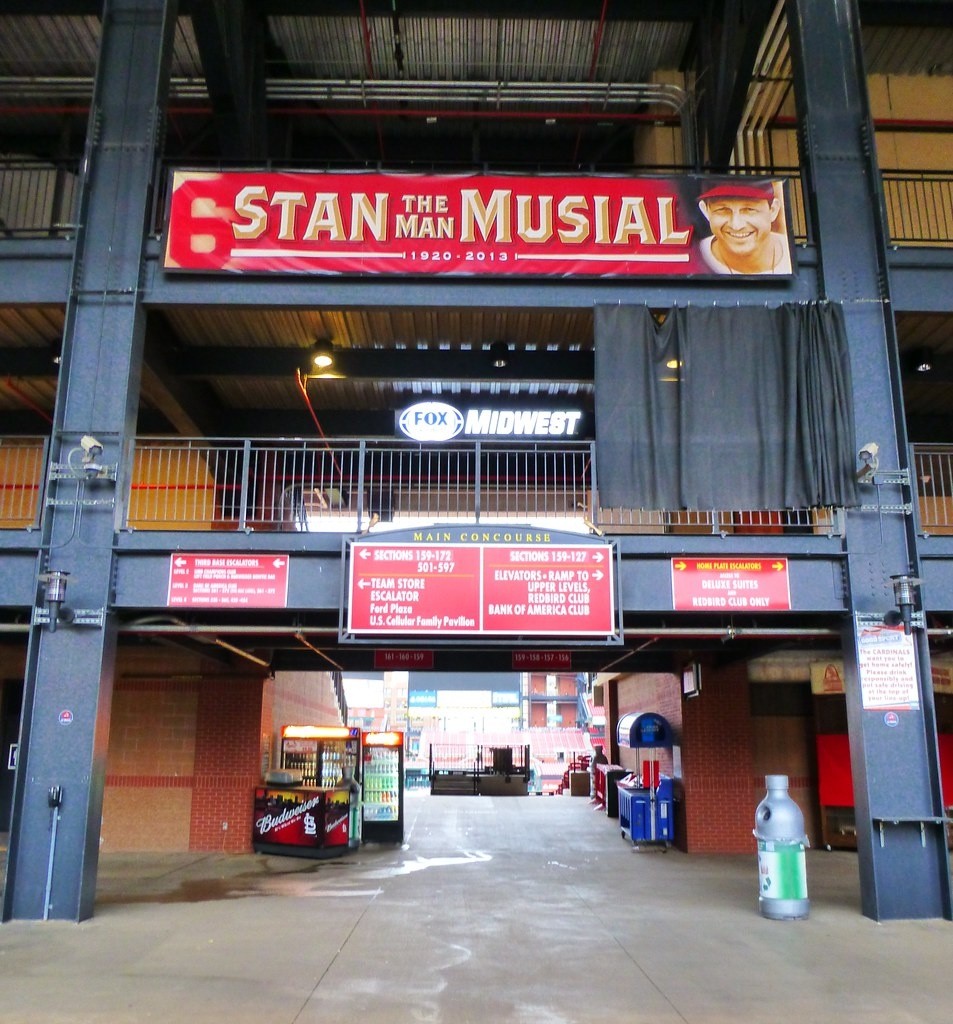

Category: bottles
[366, 792, 398, 803]
[288, 747, 316, 788]
[366, 763, 399, 774]
[335, 767, 361, 848]
[321, 741, 356, 784]
[366, 776, 398, 789]
[755, 775, 810, 921]
[365, 747, 399, 760]
[364, 806, 398, 820]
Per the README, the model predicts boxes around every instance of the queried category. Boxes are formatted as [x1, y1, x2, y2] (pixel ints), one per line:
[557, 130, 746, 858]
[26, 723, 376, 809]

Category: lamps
[914, 345, 934, 374]
[883, 572, 915, 635]
[45, 569, 75, 633]
[44, 335, 63, 373]
[311, 339, 334, 369]
[489, 341, 509, 368]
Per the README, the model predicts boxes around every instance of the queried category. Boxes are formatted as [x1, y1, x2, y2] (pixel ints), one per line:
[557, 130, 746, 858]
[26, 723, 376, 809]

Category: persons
[694, 176, 792, 274]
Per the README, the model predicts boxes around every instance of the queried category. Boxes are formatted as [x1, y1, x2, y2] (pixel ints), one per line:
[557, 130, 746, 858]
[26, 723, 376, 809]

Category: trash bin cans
[335, 765, 362, 848]
[751, 773, 812, 920]
[587, 744, 609, 800]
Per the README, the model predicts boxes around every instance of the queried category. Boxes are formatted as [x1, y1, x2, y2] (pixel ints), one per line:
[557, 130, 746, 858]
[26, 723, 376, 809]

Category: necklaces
[718, 240, 776, 274]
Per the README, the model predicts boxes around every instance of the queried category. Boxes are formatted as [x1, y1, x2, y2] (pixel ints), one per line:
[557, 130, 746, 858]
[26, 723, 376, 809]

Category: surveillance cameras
[81, 435, 103, 456]
[858, 443, 878, 460]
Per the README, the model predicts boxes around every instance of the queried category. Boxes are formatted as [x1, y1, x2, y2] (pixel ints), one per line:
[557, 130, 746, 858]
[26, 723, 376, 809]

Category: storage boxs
[568, 770, 589, 797]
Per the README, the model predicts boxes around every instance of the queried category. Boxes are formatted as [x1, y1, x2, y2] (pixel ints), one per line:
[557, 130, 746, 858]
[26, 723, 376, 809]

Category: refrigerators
[281, 725, 361, 848]
[361, 731, 403, 847]
